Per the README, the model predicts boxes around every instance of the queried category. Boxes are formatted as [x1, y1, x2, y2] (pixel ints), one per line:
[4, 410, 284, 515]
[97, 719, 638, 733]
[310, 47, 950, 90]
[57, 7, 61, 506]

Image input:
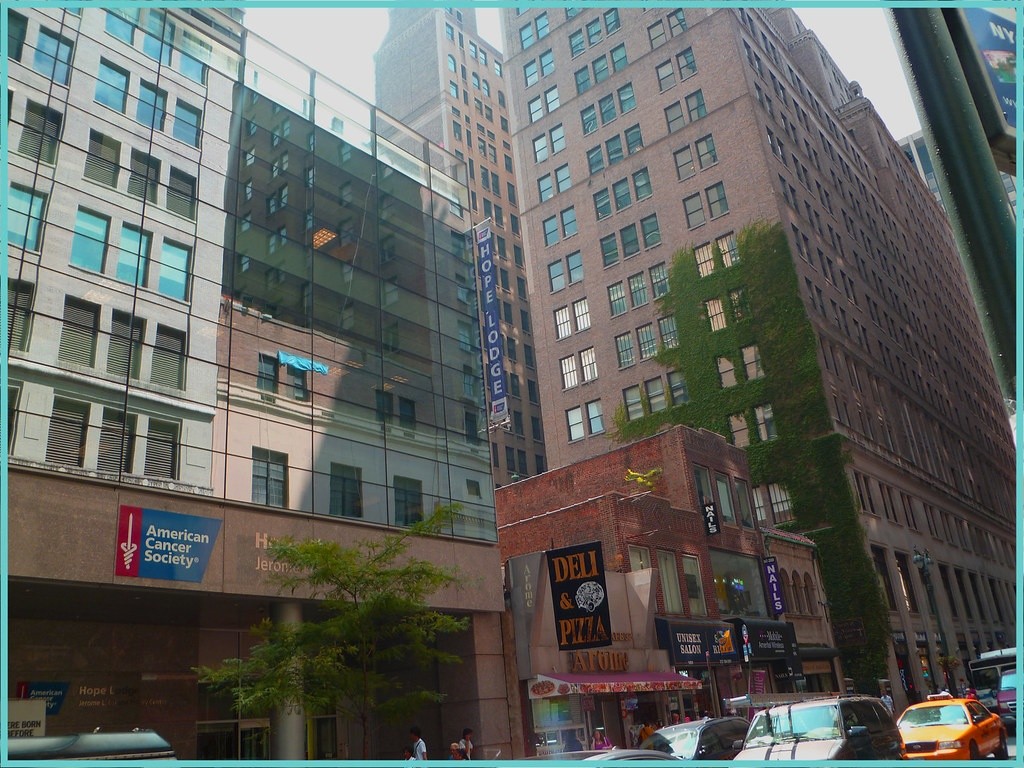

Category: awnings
[528, 673, 703, 700]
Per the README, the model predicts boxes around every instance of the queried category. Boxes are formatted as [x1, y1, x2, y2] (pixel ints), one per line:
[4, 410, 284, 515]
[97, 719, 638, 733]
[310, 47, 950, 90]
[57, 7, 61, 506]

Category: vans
[996, 668, 1016, 736]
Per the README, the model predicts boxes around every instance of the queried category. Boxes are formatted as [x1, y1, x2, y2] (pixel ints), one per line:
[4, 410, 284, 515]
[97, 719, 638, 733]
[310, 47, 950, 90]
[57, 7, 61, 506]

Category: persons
[959, 679, 976, 699]
[459, 728, 474, 760]
[410, 726, 428, 760]
[880, 689, 895, 714]
[563, 730, 584, 752]
[403, 745, 417, 760]
[590, 730, 613, 750]
[448, 742, 469, 760]
[639, 711, 710, 749]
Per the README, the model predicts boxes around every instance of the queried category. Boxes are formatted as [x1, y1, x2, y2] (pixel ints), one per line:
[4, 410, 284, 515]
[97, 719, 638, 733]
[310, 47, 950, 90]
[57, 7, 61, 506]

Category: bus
[970, 647, 1017, 714]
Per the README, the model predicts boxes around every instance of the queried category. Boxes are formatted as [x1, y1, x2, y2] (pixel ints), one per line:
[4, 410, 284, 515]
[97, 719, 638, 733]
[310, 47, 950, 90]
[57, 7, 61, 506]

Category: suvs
[732, 695, 905, 760]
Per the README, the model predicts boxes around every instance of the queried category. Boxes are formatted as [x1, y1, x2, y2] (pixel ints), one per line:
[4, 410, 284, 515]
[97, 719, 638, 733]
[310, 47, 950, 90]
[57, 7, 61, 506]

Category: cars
[640, 717, 751, 760]
[896, 694, 1008, 760]
